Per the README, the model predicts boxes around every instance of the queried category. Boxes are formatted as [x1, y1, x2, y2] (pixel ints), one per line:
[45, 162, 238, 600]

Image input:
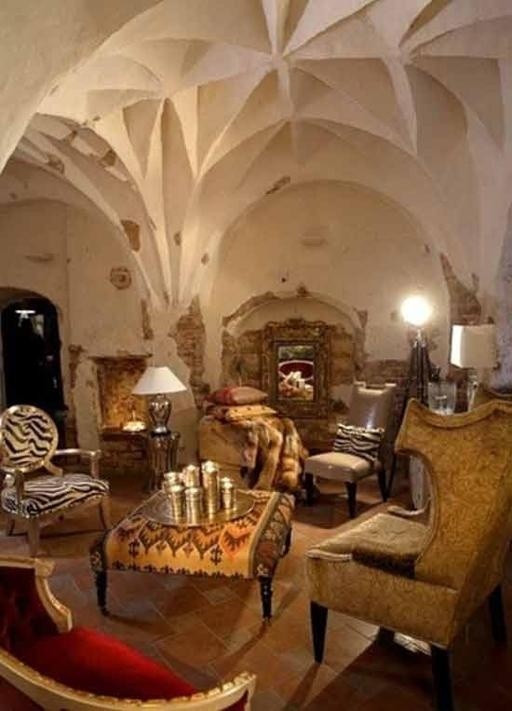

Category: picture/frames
[262, 318, 331, 420]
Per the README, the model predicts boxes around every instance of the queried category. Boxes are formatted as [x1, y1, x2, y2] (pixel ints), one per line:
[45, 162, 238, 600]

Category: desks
[127, 431, 180, 494]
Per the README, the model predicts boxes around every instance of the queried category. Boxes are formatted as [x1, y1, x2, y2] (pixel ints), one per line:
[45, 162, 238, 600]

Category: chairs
[303, 380, 398, 519]
[303, 382, 512, 711]
[0, 405, 114, 558]
[0, 551, 257, 710]
[198, 415, 309, 506]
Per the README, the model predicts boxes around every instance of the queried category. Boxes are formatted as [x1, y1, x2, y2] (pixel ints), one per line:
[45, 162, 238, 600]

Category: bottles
[162, 460, 235, 525]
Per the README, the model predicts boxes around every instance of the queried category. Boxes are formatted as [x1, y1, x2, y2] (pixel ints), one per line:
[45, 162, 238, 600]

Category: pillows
[331, 422, 384, 461]
[203, 386, 280, 421]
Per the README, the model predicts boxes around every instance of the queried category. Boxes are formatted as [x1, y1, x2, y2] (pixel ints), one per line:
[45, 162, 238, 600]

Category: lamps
[399, 293, 433, 343]
[130, 366, 187, 435]
[450, 324, 498, 410]
